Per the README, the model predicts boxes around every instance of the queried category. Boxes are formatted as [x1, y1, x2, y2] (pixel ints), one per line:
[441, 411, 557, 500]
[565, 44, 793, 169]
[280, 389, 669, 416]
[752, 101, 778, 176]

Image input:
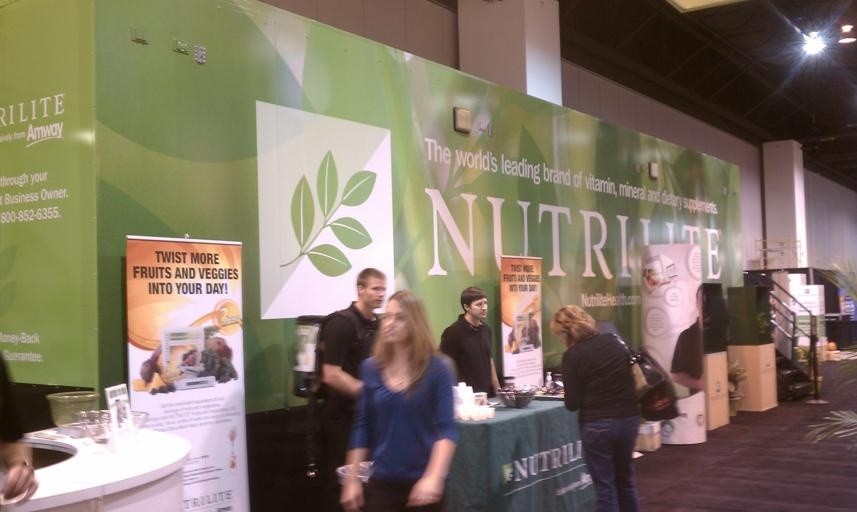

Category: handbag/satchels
[628, 348, 665, 402]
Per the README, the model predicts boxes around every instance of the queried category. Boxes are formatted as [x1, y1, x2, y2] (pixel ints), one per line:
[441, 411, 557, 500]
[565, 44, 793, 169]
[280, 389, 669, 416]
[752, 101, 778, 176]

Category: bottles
[545, 372, 563, 388]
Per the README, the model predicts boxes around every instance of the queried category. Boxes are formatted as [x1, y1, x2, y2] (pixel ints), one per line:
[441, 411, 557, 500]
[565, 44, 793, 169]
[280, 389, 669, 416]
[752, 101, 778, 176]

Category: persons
[667, 286, 707, 397]
[312, 267, 388, 510]
[440, 286, 501, 399]
[551, 304, 639, 511]
[1, 354, 39, 506]
[339, 289, 464, 512]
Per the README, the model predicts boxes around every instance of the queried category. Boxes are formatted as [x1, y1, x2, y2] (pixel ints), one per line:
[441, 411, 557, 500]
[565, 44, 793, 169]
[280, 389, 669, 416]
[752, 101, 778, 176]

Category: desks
[443, 390, 597, 512]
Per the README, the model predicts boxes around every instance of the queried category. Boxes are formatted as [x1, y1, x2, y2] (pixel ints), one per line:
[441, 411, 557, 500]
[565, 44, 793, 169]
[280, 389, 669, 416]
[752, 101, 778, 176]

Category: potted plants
[727, 360, 747, 417]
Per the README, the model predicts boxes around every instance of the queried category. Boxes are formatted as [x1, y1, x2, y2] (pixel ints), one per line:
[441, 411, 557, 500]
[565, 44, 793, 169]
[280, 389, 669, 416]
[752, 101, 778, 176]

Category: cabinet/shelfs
[702, 283, 730, 431]
[727, 287, 778, 412]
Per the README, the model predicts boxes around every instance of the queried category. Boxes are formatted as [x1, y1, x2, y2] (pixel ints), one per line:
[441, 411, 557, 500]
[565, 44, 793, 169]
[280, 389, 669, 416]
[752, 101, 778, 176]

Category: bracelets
[6, 458, 32, 469]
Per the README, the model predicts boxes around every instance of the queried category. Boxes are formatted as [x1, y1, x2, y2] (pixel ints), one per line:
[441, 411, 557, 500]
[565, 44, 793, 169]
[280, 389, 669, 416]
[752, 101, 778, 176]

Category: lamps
[839, 24, 857, 44]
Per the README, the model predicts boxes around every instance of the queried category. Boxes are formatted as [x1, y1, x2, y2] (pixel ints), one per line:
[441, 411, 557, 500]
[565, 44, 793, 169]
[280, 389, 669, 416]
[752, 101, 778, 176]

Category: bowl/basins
[78, 410, 150, 444]
[496, 392, 535, 408]
[46, 391, 101, 438]
